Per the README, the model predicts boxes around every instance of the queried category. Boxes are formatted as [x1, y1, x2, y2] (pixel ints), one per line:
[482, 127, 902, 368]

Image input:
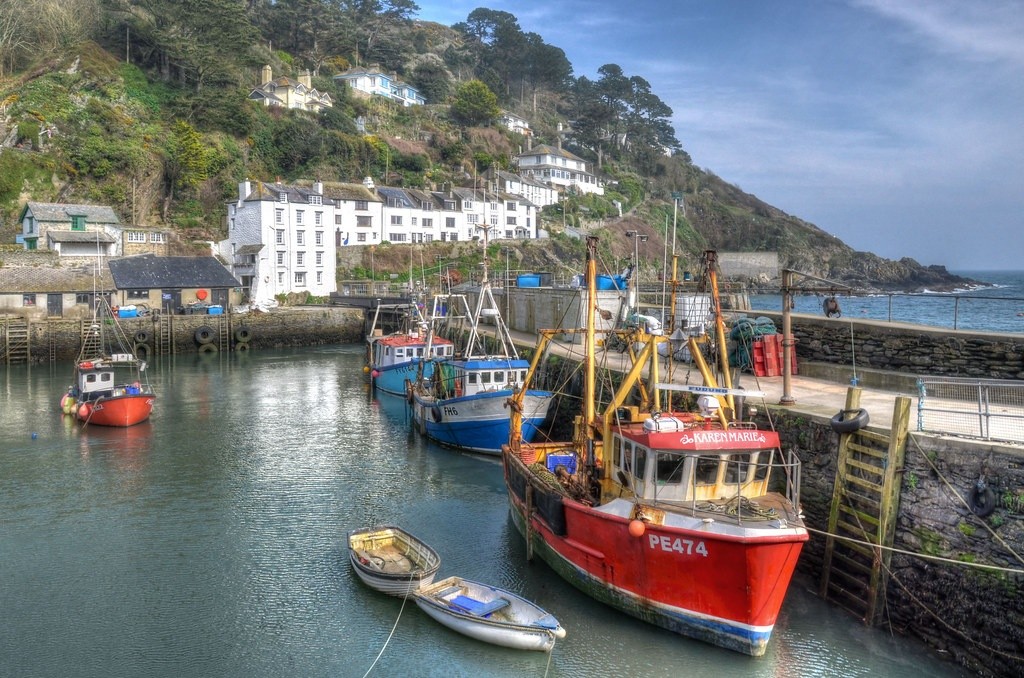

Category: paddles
[355, 547, 381, 571]
[437, 596, 475, 616]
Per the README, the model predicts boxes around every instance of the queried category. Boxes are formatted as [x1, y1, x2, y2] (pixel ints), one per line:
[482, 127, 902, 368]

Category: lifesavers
[79, 362, 93, 367]
[135, 330, 149, 342]
[967, 485, 996, 516]
[196, 289, 207, 300]
[548, 499, 567, 536]
[432, 407, 442, 422]
[236, 326, 253, 342]
[831, 408, 870, 433]
[234, 342, 250, 351]
[661, 411, 695, 423]
[199, 343, 218, 352]
[132, 343, 151, 360]
[195, 326, 214, 344]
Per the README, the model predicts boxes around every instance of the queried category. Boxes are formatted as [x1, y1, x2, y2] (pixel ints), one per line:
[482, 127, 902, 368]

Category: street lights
[626, 228, 649, 356]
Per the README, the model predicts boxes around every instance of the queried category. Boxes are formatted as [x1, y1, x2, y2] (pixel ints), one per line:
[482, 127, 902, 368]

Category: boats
[366, 293, 457, 396]
[411, 576, 567, 654]
[404, 189, 554, 456]
[500, 198, 811, 660]
[346, 525, 442, 605]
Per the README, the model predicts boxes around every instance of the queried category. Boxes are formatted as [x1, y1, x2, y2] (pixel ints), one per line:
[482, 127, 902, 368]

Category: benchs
[468, 598, 509, 616]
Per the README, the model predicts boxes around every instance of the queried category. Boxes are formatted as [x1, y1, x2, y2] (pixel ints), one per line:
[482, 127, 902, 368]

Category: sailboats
[59, 222, 156, 425]
